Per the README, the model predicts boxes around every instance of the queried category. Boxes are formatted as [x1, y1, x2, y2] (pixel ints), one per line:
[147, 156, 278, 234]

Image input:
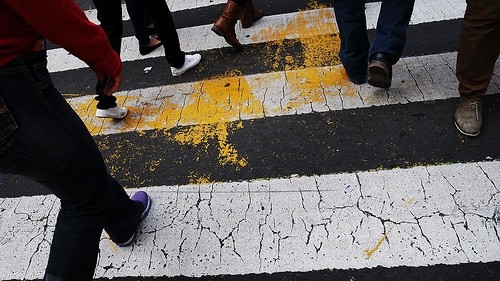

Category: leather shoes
[348, 74, 366, 84]
[368, 51, 392, 88]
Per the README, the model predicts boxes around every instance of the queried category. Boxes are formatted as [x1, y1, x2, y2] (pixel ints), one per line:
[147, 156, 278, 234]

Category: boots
[211, 0, 243, 48]
[240, 0, 264, 28]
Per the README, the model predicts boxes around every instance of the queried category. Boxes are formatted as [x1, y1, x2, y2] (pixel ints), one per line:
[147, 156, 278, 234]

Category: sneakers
[170, 53, 201, 77]
[96, 106, 128, 118]
[116, 191, 151, 247]
[455, 94, 482, 135]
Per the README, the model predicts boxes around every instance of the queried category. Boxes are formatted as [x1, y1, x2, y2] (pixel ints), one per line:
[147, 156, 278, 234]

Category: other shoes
[140, 36, 163, 55]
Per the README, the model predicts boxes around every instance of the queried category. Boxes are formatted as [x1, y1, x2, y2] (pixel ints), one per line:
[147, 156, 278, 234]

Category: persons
[125, 0, 162, 56]
[93, 0, 202, 118]
[333, 0, 416, 89]
[211, 0, 265, 50]
[0, 0, 152, 281]
[453, 0, 500, 136]
